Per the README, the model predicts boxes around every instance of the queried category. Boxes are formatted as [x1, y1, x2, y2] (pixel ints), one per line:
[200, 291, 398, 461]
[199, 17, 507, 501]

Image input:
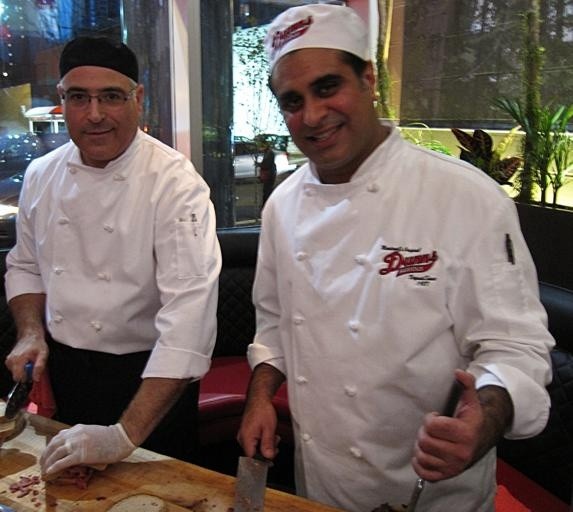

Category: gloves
[39, 422, 139, 476]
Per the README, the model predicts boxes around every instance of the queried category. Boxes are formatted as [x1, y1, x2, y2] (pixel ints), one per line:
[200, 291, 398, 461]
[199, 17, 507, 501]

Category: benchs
[0, 227, 572, 511]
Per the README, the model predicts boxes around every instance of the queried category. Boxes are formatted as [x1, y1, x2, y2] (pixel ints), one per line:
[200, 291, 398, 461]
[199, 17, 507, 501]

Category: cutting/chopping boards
[0, 398, 353, 511]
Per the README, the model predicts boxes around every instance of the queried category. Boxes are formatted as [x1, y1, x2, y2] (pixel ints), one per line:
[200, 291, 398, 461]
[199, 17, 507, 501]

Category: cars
[0, 167, 29, 243]
[231, 132, 289, 181]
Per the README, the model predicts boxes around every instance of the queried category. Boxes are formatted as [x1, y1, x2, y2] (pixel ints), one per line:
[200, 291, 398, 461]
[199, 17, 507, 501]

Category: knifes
[3, 361, 36, 421]
[233, 434, 274, 511]
[405, 378, 465, 512]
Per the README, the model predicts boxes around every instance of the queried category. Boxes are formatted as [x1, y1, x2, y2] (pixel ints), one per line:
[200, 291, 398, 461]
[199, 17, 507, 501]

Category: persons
[236, 2, 557, 512]
[5, 36, 222, 478]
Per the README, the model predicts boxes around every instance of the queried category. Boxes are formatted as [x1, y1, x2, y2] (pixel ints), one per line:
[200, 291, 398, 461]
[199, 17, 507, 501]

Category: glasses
[58, 86, 141, 111]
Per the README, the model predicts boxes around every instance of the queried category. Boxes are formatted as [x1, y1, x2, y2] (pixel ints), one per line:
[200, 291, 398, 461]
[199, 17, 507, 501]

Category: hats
[59, 36, 138, 85]
[265, 2, 374, 77]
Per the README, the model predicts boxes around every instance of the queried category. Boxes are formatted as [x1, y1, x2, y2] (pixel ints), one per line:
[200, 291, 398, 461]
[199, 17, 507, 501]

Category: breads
[103, 493, 164, 512]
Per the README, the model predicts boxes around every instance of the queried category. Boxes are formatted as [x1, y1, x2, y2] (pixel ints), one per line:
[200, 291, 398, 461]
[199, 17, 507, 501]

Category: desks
[0, 397, 348, 511]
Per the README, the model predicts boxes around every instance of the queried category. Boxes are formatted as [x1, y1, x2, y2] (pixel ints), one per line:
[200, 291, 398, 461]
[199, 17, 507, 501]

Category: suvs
[0, 127, 70, 170]
